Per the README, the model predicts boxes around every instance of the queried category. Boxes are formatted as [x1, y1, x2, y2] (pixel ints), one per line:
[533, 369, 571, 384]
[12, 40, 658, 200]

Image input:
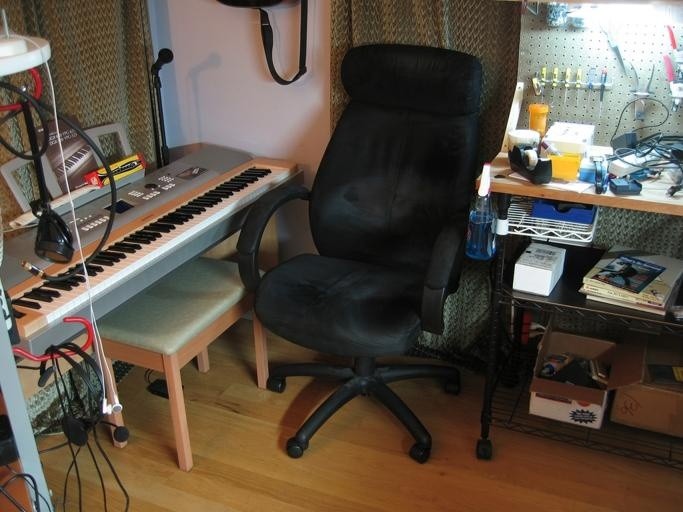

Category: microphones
[150, 47, 174, 75]
[100, 418, 132, 443]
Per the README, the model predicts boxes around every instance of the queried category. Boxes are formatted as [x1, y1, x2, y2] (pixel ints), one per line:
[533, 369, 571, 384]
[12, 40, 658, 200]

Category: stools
[89, 251, 274, 472]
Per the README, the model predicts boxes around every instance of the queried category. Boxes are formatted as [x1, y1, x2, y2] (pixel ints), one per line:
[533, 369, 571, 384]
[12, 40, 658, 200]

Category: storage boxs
[606, 327, 683, 442]
[524, 305, 653, 438]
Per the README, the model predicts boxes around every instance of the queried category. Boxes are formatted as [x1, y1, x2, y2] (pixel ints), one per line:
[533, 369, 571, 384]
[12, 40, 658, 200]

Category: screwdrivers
[541, 67, 608, 118]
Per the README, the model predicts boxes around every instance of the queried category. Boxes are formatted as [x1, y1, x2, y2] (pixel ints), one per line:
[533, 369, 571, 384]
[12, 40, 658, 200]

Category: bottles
[540, 351, 574, 377]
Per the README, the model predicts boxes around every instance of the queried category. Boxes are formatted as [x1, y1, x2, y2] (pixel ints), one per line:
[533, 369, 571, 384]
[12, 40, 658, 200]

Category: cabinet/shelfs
[468, 135, 683, 475]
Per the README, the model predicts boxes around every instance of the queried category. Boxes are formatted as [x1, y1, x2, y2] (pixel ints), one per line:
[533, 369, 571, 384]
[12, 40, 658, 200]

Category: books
[578, 243, 683, 320]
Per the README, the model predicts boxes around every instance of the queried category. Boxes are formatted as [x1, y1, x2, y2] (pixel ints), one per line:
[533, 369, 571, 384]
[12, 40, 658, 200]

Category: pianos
[5, 155, 299, 338]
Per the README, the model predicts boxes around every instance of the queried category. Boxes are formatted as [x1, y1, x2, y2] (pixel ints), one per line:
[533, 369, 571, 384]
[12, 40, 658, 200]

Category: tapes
[524, 149, 538, 171]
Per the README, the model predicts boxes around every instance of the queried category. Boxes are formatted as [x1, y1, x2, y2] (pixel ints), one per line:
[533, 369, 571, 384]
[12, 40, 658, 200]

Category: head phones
[38, 342, 103, 447]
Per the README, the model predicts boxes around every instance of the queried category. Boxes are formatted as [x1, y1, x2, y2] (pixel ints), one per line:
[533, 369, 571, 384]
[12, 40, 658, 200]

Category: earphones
[110, 393, 125, 414]
[101, 397, 113, 418]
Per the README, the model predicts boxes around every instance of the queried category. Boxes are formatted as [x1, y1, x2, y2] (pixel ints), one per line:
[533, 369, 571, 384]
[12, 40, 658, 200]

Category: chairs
[225, 40, 488, 466]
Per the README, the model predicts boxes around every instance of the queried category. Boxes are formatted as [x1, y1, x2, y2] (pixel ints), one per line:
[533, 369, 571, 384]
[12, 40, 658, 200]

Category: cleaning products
[465, 162, 498, 261]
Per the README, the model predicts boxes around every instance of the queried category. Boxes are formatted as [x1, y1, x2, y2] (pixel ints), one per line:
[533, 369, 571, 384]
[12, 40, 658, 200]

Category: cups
[528, 101, 549, 134]
[508, 129, 540, 152]
[545, 2, 567, 28]
